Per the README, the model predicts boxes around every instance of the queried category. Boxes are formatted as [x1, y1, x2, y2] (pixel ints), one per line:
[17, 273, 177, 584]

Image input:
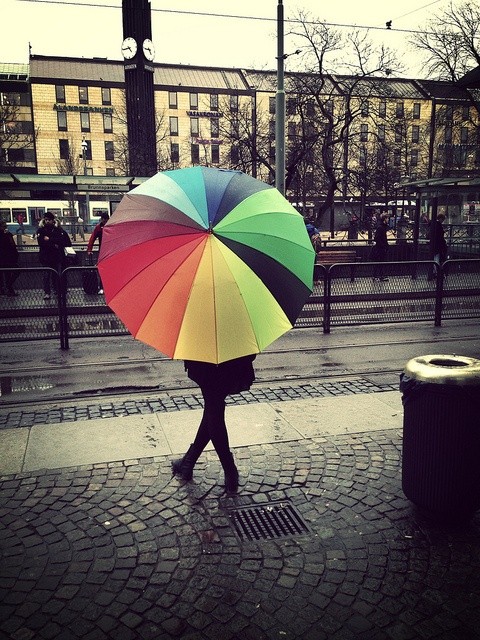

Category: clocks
[122, 35, 137, 60]
[141, 40, 155, 61]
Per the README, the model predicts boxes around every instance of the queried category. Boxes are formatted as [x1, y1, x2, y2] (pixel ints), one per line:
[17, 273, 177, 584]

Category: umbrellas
[95, 165, 318, 369]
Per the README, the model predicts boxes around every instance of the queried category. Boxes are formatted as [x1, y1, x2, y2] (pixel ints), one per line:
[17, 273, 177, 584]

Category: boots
[213, 450, 249, 494]
[162, 439, 202, 483]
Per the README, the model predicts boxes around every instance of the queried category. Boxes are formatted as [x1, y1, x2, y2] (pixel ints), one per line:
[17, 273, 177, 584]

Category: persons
[172, 352, 256, 495]
[368, 211, 376, 233]
[348, 215, 359, 239]
[37, 213, 60, 228]
[393, 215, 396, 229]
[428, 214, 451, 281]
[15, 213, 26, 235]
[0, 220, 22, 297]
[420, 211, 427, 238]
[303, 219, 322, 285]
[396, 213, 410, 242]
[36, 212, 72, 300]
[372, 215, 391, 283]
[87, 213, 111, 295]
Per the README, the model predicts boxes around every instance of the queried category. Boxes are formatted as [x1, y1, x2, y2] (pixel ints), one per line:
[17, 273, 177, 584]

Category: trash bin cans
[399, 354, 477, 508]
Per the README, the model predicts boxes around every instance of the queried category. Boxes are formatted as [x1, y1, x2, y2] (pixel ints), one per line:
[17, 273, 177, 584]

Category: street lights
[272, 3, 305, 280]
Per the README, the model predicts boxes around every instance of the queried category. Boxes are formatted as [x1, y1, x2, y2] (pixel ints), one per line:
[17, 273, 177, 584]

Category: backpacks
[309, 227, 322, 252]
